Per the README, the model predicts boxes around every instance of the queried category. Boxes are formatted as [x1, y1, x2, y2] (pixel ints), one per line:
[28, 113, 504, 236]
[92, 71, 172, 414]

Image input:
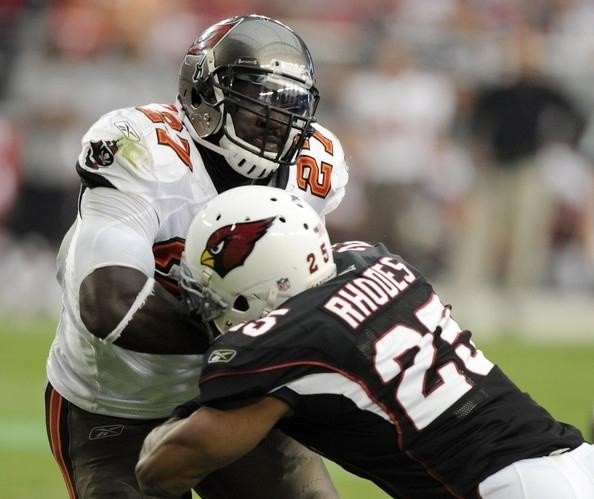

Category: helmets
[184, 183, 336, 336]
[176, 15, 319, 180]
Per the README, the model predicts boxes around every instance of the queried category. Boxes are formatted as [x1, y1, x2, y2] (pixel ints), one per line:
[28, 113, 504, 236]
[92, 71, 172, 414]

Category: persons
[135, 183, 594, 499]
[43, 14, 350, 498]
